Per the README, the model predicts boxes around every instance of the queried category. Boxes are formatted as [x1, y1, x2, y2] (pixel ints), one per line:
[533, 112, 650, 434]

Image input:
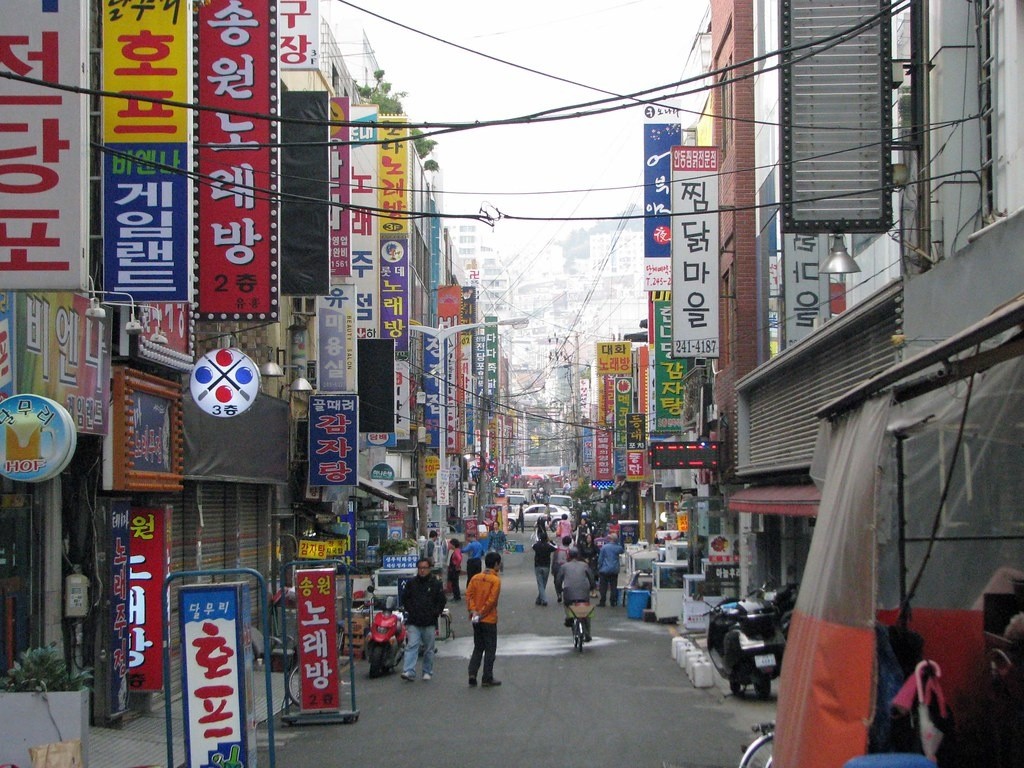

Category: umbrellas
[915, 661, 942, 768]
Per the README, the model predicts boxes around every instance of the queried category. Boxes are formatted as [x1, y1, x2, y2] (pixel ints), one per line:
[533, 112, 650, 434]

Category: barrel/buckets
[626, 589, 650, 618]
[479, 538, 488, 556]
[671, 635, 713, 687]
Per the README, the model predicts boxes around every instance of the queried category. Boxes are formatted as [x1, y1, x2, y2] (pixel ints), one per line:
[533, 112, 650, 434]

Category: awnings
[728, 485, 821, 516]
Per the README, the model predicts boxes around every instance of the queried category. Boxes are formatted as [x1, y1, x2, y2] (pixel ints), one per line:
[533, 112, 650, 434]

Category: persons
[573, 518, 590, 551]
[551, 536, 571, 602]
[555, 547, 594, 642]
[653, 526, 665, 544]
[533, 516, 547, 540]
[533, 533, 557, 606]
[515, 503, 525, 533]
[948, 610, 1024, 768]
[422, 531, 438, 567]
[466, 553, 501, 686]
[597, 535, 625, 608]
[400, 558, 448, 681]
[448, 539, 463, 602]
[461, 532, 485, 588]
[555, 514, 572, 542]
[582, 534, 600, 598]
[488, 521, 506, 575]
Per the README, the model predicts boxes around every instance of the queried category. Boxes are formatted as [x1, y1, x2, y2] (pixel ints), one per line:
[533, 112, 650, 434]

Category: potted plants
[377, 538, 417, 555]
[0, 645, 95, 768]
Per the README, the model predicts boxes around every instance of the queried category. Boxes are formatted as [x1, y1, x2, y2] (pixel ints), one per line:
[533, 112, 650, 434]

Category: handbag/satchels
[556, 522, 561, 537]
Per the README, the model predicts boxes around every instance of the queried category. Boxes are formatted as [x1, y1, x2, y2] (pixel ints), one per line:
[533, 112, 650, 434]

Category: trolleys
[277, 558, 360, 727]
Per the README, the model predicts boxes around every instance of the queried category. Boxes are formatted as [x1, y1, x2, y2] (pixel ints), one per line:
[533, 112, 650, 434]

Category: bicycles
[736, 721, 775, 768]
[288, 595, 353, 708]
[557, 588, 598, 653]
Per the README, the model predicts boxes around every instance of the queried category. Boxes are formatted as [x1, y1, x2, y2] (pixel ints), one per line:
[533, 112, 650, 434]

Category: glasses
[417, 566, 429, 570]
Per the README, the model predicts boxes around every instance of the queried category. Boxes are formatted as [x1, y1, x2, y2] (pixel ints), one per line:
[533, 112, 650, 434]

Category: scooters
[690, 586, 786, 700]
[757, 565, 801, 640]
[363, 586, 408, 681]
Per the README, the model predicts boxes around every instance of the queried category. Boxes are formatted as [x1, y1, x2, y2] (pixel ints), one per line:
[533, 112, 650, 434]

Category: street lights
[408, 316, 530, 558]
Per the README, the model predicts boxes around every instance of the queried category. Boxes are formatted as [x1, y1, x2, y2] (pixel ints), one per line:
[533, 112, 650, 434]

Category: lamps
[242, 346, 284, 377]
[87, 291, 143, 335]
[258, 364, 313, 392]
[101, 302, 168, 346]
[85, 275, 105, 322]
[819, 234, 861, 275]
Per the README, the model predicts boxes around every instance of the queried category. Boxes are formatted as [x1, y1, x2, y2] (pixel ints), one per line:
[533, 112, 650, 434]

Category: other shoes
[482, 679, 502, 686]
[596, 604, 605, 607]
[422, 674, 431, 681]
[565, 618, 574, 627]
[536, 600, 541, 605]
[468, 675, 477, 685]
[401, 672, 414, 681]
[590, 593, 598, 598]
[543, 601, 547, 606]
[584, 635, 592, 642]
[611, 602, 617, 607]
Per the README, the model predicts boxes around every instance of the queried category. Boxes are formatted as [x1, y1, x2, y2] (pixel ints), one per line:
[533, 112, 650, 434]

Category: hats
[609, 533, 618, 542]
[569, 546, 578, 558]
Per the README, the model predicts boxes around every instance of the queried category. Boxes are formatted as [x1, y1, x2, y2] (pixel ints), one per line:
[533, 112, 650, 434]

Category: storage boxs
[344, 618, 369, 657]
[738, 599, 776, 634]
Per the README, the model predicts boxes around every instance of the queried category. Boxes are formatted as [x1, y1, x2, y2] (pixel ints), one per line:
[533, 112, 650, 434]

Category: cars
[506, 488, 589, 533]
